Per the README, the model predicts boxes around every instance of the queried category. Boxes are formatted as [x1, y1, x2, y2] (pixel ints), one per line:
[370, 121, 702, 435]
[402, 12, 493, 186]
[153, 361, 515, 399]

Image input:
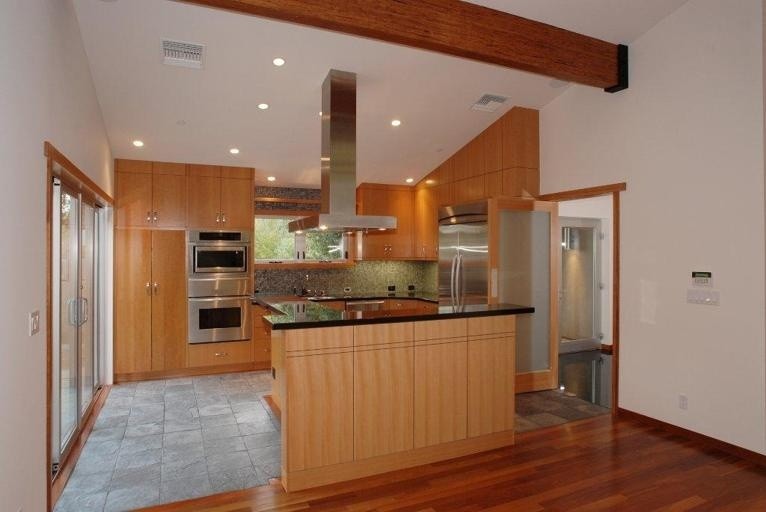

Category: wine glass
[344, 282, 352, 299]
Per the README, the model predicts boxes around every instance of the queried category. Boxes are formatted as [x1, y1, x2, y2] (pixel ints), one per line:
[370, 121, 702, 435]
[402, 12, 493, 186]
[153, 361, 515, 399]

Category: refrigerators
[439, 211, 550, 368]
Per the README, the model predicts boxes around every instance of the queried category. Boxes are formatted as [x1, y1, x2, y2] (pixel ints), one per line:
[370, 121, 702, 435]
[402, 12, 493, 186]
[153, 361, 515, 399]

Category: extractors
[288, 69, 398, 233]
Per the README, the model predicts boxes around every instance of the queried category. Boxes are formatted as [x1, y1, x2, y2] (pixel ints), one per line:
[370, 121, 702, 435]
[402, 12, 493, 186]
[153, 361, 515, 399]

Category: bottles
[292, 271, 321, 296]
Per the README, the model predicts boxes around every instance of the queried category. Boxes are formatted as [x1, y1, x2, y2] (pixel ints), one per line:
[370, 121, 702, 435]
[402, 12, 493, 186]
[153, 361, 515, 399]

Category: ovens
[187, 230, 252, 345]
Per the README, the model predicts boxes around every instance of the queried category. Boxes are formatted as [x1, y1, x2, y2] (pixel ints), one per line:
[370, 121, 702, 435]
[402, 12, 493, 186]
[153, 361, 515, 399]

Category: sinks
[305, 295, 337, 300]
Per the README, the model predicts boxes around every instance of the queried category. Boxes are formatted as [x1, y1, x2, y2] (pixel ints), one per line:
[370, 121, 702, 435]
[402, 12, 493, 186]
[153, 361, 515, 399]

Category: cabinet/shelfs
[113, 157, 187, 382]
[414, 167, 439, 261]
[186, 163, 256, 230]
[439, 106, 539, 225]
[254, 303, 273, 370]
[356, 183, 414, 261]
[312, 299, 438, 320]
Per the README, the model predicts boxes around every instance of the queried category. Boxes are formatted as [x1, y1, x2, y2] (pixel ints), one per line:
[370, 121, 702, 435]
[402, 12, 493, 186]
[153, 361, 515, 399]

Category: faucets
[313, 289, 318, 296]
